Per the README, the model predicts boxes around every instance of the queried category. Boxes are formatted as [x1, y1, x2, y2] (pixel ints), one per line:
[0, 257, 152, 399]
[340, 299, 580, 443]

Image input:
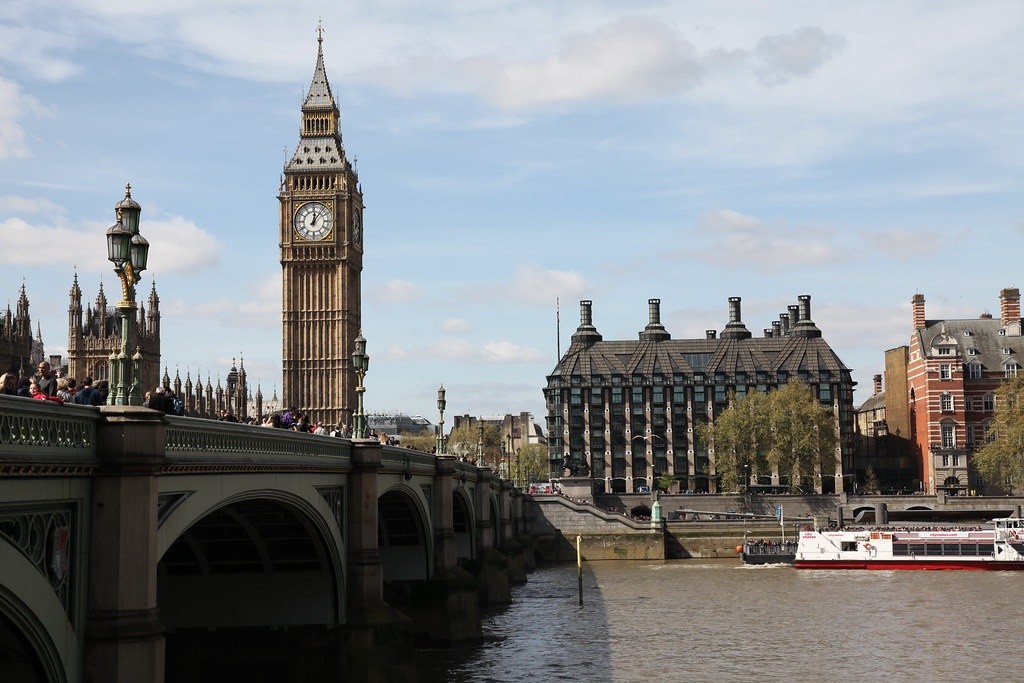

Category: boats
[736, 505, 1024, 571]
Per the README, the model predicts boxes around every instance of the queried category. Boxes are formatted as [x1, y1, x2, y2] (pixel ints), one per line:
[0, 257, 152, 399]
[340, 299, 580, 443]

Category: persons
[530, 485, 586, 504]
[143, 386, 185, 416]
[803, 523, 983, 535]
[369, 428, 436, 453]
[747, 538, 798, 554]
[446, 449, 477, 466]
[751, 488, 791, 496]
[631, 512, 649, 522]
[661, 488, 709, 496]
[217, 407, 352, 439]
[774, 503, 782, 521]
[607, 504, 621, 513]
[0, 361, 109, 405]
[709, 511, 742, 520]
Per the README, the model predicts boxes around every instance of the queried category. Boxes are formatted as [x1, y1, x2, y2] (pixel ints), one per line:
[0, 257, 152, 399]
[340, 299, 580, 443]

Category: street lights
[104, 182, 151, 408]
[437, 383, 446, 454]
[498, 439, 506, 479]
[515, 446, 521, 487]
[352, 329, 369, 439]
[477, 414, 485, 466]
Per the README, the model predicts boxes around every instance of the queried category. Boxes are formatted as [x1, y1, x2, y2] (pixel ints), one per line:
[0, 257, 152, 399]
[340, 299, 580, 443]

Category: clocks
[353, 207, 360, 245]
[293, 201, 333, 241]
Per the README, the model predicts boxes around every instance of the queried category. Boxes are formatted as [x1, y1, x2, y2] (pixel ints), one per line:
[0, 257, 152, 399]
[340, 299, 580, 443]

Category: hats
[56, 378, 69, 388]
[156, 386, 165, 394]
[317, 421, 322, 425]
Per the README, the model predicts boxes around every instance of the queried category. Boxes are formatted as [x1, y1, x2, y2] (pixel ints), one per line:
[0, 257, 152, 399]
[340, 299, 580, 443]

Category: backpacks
[173, 396, 181, 413]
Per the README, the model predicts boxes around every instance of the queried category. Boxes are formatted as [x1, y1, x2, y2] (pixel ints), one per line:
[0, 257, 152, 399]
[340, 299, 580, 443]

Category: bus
[747, 483, 805, 496]
